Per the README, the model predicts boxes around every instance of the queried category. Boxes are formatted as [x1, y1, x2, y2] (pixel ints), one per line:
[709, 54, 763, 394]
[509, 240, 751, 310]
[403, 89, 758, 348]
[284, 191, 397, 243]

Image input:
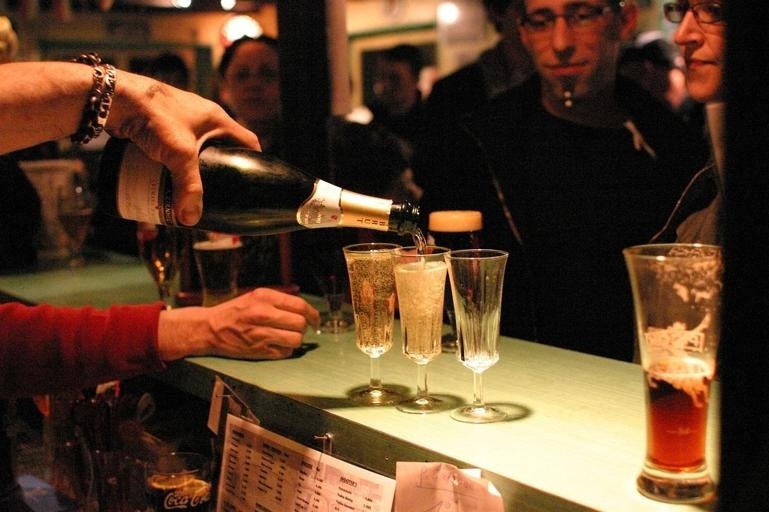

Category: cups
[142, 450, 213, 511]
[624, 243, 723, 505]
[191, 228, 244, 308]
[428, 211, 483, 354]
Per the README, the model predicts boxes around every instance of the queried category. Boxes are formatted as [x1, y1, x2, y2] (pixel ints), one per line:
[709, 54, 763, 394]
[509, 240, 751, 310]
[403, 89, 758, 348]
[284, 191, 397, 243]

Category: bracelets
[68, 53, 117, 145]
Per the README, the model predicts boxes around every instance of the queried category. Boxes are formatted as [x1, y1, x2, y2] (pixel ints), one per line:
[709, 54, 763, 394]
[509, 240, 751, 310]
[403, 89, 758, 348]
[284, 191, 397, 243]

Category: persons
[0, 17, 43, 275]
[363, 44, 425, 152]
[218, 34, 282, 151]
[420, 0, 705, 353]
[0, 60, 322, 397]
[420, 1, 530, 225]
[634, 0, 723, 366]
[91, 51, 189, 258]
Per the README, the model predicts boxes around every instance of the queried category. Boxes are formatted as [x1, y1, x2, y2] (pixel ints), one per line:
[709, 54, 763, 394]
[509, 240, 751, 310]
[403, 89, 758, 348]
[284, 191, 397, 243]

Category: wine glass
[135, 222, 183, 314]
[445, 248, 508, 423]
[390, 244, 446, 415]
[56, 170, 93, 270]
[300, 162, 366, 334]
[343, 242, 402, 406]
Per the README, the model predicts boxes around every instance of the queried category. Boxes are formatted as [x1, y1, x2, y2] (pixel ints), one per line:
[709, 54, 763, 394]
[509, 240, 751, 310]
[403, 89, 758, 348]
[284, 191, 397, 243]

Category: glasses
[662, 1, 726, 24]
[522, 0, 623, 33]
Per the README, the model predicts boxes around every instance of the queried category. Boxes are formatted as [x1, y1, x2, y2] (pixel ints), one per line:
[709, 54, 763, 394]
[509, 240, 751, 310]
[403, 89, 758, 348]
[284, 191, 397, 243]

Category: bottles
[56, 441, 82, 506]
[93, 134, 421, 239]
